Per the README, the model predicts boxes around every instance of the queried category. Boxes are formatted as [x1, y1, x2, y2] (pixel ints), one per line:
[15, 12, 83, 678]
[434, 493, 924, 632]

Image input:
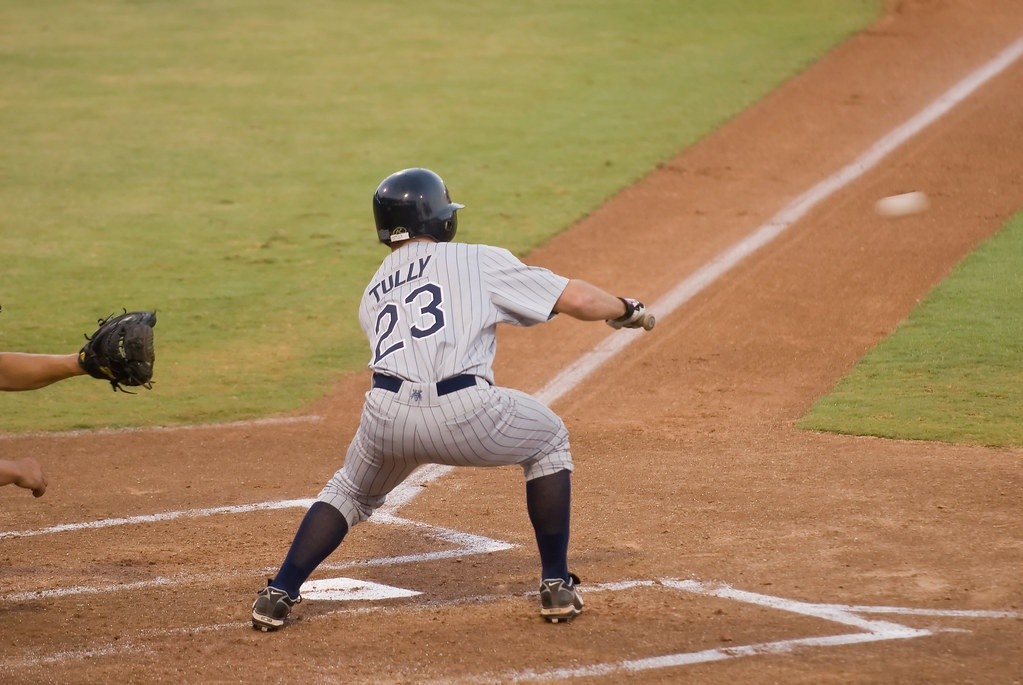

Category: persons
[252, 168, 647, 633]
[0, 308, 157, 498]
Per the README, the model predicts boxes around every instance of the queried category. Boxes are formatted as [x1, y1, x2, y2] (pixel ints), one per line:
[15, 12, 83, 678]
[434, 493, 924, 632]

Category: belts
[372, 374, 492, 396]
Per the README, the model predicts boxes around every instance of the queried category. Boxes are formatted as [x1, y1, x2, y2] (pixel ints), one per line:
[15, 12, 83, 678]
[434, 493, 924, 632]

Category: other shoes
[252, 578, 302, 631]
[539, 572, 583, 623]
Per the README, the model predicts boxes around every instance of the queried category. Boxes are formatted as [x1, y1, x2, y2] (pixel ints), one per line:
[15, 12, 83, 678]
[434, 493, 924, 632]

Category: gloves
[605, 296, 646, 330]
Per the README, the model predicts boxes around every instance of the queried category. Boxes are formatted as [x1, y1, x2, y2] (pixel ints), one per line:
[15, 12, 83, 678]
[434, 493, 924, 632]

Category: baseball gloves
[80, 312, 157, 386]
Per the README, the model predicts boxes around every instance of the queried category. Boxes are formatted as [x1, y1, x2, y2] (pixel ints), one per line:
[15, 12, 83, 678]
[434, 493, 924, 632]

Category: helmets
[373, 168, 466, 243]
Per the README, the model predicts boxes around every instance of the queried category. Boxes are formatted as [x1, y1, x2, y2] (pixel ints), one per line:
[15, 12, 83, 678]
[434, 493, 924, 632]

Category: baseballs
[875, 189, 930, 217]
